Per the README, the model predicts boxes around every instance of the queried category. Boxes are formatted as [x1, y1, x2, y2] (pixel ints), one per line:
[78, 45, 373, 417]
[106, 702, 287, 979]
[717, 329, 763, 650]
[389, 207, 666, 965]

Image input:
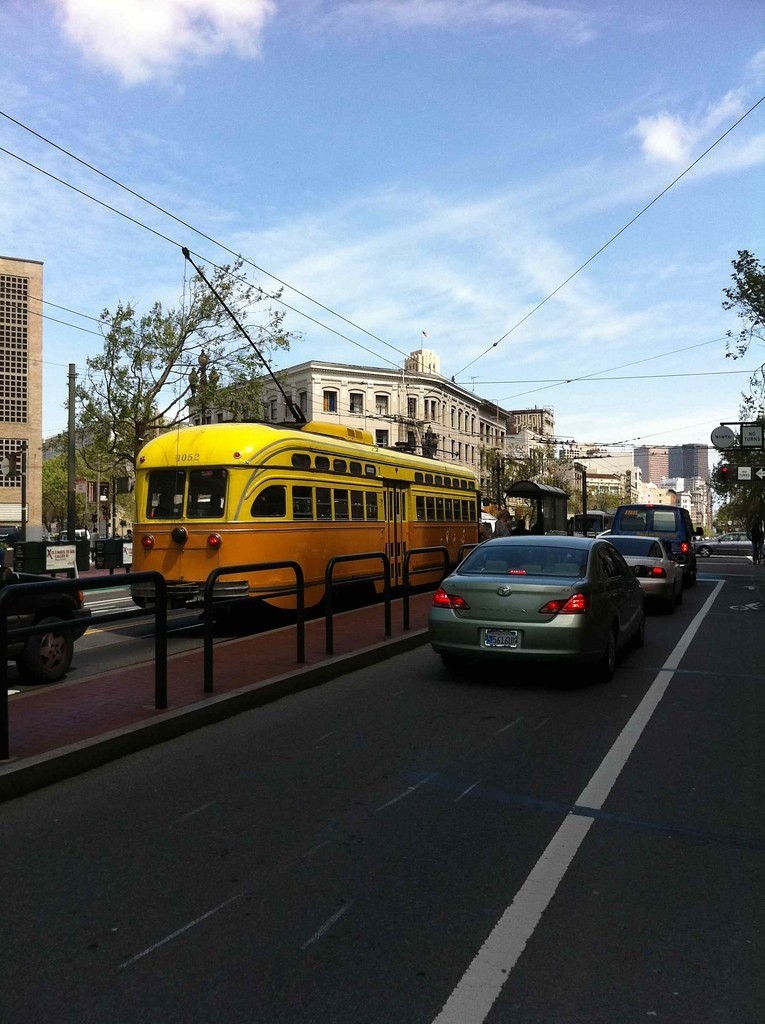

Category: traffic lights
[720, 464, 729, 480]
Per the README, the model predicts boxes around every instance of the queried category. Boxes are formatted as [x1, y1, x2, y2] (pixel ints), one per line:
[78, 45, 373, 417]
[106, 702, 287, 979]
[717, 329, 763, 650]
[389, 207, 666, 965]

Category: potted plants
[0, 542, 14, 567]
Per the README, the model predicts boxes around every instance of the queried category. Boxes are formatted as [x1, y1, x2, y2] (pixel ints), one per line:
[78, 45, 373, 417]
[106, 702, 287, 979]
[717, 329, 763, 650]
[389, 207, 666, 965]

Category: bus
[128, 421, 490, 624]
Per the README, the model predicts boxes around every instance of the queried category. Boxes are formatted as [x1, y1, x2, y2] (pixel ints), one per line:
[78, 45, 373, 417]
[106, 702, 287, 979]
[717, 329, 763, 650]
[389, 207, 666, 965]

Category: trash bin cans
[95, 537, 133, 567]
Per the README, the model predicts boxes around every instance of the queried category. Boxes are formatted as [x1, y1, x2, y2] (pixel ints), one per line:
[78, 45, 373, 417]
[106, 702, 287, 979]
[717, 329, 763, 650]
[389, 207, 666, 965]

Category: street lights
[187, 348, 220, 424]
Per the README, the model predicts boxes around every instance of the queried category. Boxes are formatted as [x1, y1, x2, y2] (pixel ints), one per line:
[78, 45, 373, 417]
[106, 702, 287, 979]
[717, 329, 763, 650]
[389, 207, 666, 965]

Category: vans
[60, 529, 91, 541]
[611, 504, 704, 589]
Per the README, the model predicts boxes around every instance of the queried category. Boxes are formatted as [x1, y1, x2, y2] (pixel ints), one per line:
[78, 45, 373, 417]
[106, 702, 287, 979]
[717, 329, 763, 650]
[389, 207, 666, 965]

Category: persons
[89, 527, 99, 562]
[478, 510, 537, 542]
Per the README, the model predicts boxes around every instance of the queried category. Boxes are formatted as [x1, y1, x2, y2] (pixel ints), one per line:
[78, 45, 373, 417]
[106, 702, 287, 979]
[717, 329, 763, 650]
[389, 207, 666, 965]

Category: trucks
[570, 510, 608, 539]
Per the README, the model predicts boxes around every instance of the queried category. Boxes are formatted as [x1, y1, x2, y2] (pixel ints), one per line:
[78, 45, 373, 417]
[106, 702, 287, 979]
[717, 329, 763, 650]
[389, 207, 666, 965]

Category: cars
[595, 535, 687, 616]
[427, 535, 647, 685]
[694, 531, 765, 560]
[0, 563, 93, 687]
